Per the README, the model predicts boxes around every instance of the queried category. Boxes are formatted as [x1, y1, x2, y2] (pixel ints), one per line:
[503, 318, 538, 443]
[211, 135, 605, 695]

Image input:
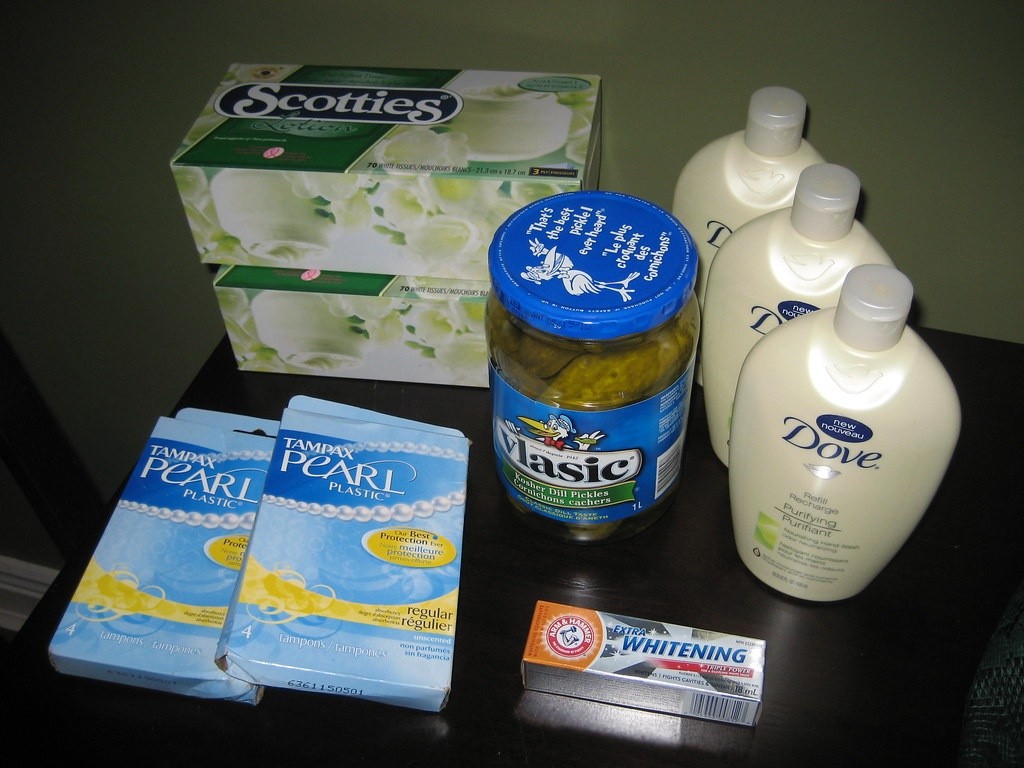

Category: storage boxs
[211, 264, 493, 388]
[520, 600, 768, 727]
[44, 406, 281, 706]
[167, 61, 604, 282]
[213, 393, 473, 712]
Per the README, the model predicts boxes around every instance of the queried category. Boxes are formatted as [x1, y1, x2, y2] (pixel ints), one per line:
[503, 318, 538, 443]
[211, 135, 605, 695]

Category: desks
[0, 322, 1024, 768]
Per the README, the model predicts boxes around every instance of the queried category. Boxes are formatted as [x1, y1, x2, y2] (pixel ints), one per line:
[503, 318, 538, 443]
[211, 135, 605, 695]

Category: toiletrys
[702, 161, 900, 471]
[675, 85, 830, 390]
[726, 263, 964, 604]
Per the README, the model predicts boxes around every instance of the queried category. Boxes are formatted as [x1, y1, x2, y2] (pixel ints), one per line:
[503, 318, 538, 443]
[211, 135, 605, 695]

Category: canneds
[484, 194, 701, 544]
